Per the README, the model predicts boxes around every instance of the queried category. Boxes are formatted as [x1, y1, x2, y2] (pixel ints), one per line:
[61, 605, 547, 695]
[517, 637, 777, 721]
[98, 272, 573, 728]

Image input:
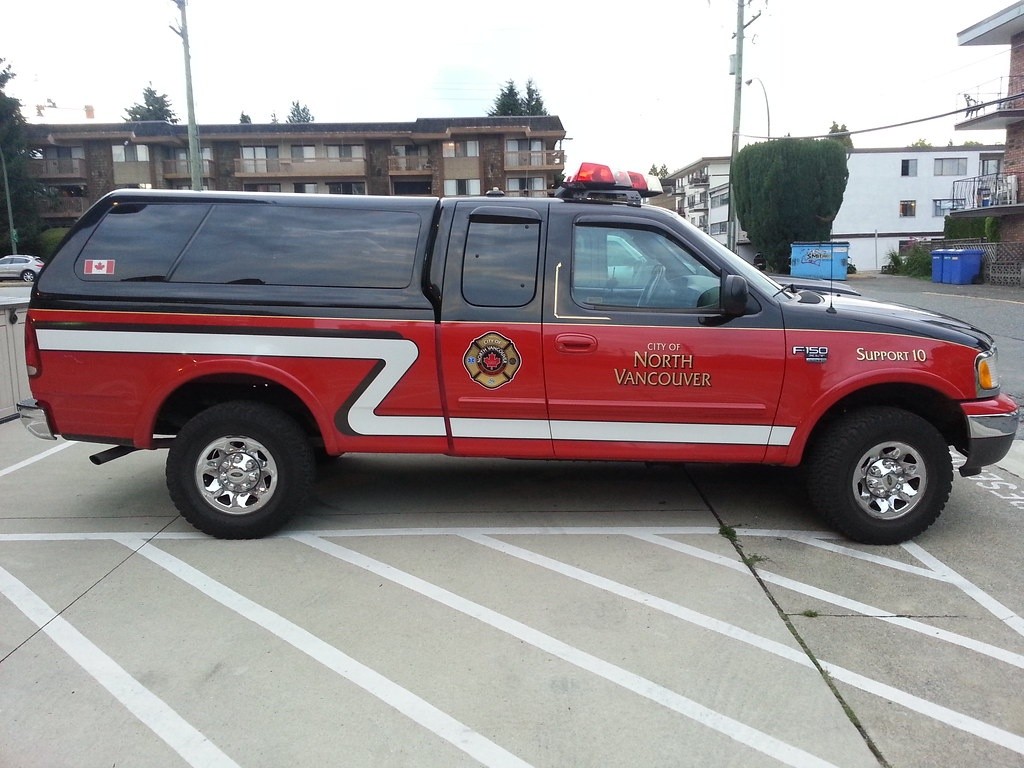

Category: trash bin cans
[932, 248, 986, 284]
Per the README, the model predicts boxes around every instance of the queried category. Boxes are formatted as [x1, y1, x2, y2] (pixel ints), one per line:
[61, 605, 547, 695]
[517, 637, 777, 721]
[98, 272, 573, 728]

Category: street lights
[746, 75, 771, 144]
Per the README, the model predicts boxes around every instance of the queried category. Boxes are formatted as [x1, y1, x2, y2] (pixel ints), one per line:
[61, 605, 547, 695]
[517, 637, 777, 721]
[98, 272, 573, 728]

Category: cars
[606, 236, 647, 289]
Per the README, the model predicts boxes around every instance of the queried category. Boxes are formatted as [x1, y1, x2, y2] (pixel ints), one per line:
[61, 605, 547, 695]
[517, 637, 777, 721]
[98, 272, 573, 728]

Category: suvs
[0, 254, 43, 285]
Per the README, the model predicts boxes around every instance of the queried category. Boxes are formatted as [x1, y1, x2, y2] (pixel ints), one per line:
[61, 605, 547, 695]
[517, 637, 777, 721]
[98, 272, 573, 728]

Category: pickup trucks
[15, 164, 1017, 548]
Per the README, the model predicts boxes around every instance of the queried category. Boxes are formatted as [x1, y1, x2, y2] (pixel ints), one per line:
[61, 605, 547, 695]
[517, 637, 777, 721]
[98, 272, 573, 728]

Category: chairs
[991, 175, 1019, 204]
